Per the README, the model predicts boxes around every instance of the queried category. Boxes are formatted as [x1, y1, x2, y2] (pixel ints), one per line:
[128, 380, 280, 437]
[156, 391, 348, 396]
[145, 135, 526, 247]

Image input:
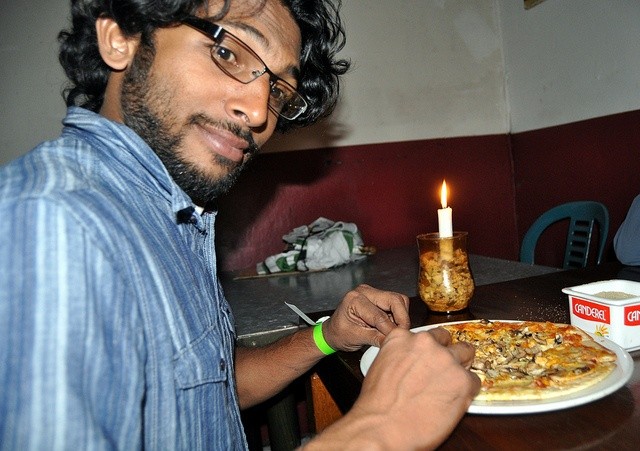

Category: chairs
[519, 199, 610, 267]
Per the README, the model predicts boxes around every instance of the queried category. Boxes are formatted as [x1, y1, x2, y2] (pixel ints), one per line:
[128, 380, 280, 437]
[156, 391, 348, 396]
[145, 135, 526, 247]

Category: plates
[359, 320, 633, 414]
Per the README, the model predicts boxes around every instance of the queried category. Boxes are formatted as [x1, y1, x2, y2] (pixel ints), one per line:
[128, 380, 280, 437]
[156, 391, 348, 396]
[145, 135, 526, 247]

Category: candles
[437, 177, 455, 260]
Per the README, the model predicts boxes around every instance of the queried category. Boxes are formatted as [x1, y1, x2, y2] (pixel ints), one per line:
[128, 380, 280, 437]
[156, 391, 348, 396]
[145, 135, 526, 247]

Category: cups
[417, 232, 475, 314]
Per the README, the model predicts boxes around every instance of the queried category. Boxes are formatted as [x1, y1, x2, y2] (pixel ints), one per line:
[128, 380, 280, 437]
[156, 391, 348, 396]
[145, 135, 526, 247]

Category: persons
[0, 0, 484, 451]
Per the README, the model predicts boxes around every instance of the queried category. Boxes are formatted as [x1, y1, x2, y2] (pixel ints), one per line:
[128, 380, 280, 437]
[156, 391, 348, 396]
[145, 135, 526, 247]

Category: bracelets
[312, 315, 338, 356]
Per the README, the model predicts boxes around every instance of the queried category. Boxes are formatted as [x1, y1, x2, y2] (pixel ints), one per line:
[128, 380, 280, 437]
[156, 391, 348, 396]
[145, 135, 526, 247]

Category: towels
[255, 216, 377, 273]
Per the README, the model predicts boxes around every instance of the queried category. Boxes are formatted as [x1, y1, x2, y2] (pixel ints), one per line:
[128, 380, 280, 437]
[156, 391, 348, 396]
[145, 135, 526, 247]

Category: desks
[217, 231, 568, 450]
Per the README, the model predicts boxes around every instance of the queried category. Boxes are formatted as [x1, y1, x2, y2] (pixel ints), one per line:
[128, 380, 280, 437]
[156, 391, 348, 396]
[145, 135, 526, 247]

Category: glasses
[179, 15, 311, 121]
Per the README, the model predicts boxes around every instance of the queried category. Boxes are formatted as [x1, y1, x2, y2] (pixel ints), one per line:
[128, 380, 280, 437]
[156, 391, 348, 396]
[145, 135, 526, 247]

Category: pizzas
[435, 318, 618, 402]
